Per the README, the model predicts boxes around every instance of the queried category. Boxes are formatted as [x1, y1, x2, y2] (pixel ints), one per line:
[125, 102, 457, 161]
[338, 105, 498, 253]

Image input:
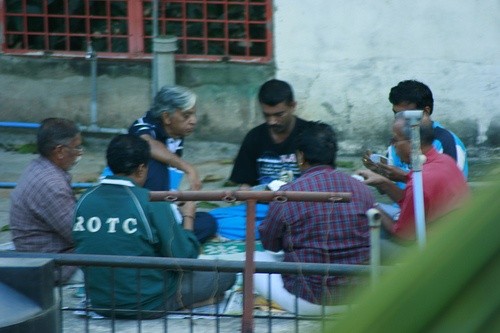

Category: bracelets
[181, 214, 196, 220]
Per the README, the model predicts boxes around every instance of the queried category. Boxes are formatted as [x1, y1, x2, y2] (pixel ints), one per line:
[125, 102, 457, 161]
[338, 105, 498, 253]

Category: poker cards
[370, 154, 390, 165]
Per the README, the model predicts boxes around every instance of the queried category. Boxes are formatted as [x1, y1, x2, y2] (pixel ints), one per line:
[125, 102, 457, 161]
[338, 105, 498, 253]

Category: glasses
[62, 144, 85, 154]
[390, 138, 410, 147]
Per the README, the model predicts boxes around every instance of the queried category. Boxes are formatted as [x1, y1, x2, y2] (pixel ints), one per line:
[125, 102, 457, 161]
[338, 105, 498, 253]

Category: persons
[238, 119, 376, 322]
[362, 79, 469, 225]
[7, 116, 87, 287]
[98, 85, 218, 246]
[202, 78, 325, 244]
[351, 109, 474, 263]
[69, 134, 237, 320]
